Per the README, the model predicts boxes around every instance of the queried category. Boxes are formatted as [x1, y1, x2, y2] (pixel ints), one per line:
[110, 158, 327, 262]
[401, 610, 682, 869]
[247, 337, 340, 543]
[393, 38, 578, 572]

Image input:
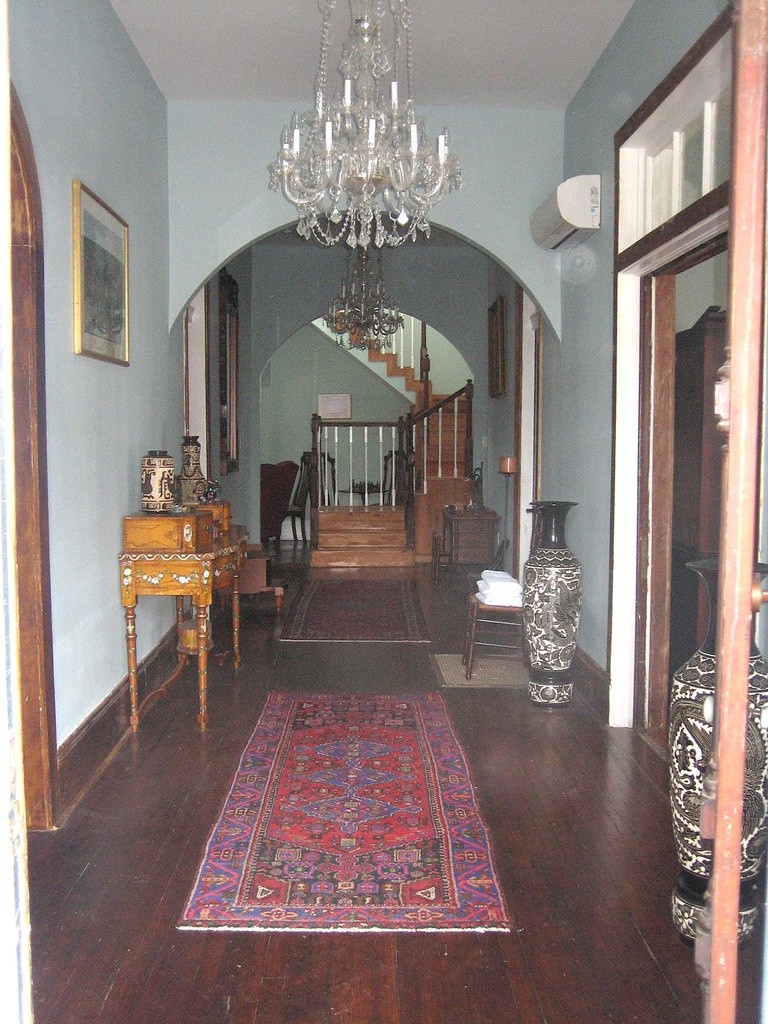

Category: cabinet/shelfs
[441, 506, 496, 569]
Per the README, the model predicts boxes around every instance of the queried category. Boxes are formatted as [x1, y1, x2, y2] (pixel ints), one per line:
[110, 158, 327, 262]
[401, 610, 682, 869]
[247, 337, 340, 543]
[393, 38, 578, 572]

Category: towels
[474, 569, 525, 608]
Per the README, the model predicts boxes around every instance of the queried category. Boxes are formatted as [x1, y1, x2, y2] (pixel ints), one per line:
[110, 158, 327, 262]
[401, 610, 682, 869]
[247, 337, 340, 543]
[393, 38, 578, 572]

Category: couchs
[260, 461, 300, 554]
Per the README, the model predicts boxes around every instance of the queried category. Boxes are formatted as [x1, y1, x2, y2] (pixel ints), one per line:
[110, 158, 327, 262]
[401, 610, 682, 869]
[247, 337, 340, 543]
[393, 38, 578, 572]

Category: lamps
[329, 238, 404, 350]
[713, 343, 731, 452]
[270, 0, 463, 250]
[498, 456, 517, 573]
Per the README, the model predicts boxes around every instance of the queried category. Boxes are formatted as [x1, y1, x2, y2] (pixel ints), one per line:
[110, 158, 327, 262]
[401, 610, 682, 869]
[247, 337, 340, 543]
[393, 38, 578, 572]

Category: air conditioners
[529, 174, 602, 255]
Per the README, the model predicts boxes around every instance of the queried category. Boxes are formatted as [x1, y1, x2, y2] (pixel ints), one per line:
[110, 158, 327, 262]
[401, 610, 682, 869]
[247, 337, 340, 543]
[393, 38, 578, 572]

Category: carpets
[277, 576, 432, 644]
[175, 691, 514, 932]
[428, 653, 531, 688]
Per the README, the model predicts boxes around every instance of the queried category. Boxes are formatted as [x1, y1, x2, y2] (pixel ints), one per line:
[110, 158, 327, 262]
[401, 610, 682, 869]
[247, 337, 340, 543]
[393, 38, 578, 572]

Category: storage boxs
[122, 510, 214, 552]
[195, 500, 230, 531]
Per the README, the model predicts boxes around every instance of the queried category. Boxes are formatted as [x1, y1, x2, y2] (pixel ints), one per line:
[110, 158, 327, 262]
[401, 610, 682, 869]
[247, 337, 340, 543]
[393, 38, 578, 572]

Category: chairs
[430, 532, 452, 583]
[462, 509, 545, 679]
[466, 539, 509, 597]
[284, 452, 312, 544]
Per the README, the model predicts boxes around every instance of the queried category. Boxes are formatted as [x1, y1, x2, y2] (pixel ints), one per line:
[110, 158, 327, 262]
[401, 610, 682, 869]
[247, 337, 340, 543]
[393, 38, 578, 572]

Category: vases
[521, 500, 582, 708]
[667, 555, 768, 950]
[177, 435, 208, 505]
[141, 450, 174, 513]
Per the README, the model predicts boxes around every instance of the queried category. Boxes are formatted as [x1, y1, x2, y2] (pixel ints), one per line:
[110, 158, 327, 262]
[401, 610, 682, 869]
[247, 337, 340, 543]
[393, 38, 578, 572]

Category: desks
[118, 525, 250, 731]
[339, 488, 387, 506]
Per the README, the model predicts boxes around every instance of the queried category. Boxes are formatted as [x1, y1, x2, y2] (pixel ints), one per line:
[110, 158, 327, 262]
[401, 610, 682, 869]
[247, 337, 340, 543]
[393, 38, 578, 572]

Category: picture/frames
[218, 267, 240, 476]
[487, 296, 506, 398]
[72, 177, 130, 366]
[317, 392, 352, 419]
